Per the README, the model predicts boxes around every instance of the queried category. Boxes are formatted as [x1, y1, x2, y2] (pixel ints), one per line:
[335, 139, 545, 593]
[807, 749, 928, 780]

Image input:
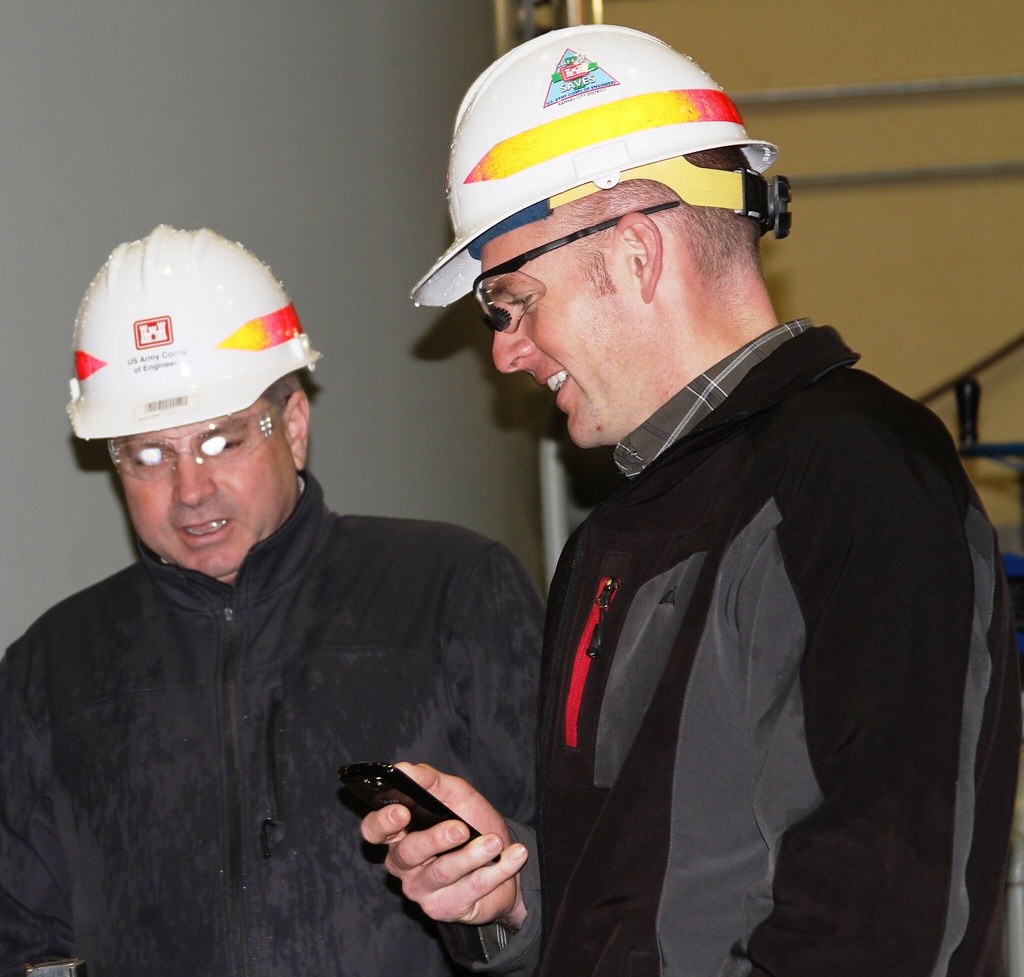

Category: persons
[363, 21, 1015, 977]
[3, 222, 540, 977]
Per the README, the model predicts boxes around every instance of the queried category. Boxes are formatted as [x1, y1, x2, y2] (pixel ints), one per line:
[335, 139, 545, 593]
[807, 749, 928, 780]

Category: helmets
[409, 23, 794, 307]
[66, 225, 322, 442]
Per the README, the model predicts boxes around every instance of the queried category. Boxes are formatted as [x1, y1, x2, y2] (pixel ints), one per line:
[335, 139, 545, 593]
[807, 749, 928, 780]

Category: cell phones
[336, 763, 500, 866]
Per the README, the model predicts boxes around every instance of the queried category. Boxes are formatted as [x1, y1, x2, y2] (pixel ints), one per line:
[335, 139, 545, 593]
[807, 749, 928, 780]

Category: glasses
[106, 397, 297, 483]
[468, 201, 682, 335]
